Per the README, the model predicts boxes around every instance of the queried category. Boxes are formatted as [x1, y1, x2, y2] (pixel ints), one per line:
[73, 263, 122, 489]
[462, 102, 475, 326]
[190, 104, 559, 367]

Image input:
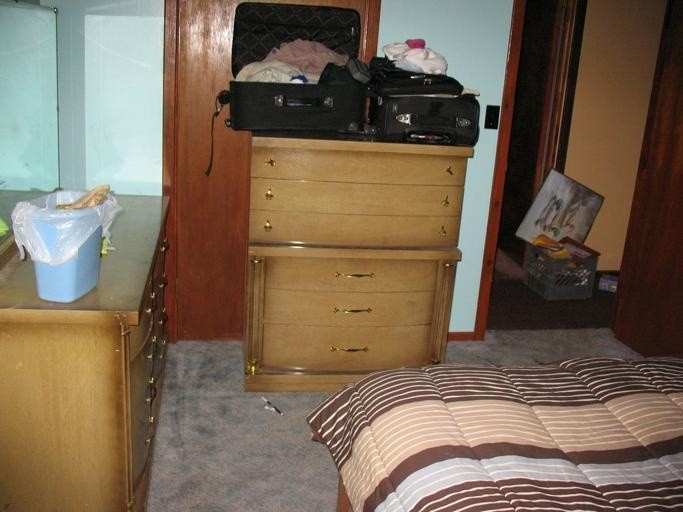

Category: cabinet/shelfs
[1, 217, 173, 511]
[242, 135, 475, 395]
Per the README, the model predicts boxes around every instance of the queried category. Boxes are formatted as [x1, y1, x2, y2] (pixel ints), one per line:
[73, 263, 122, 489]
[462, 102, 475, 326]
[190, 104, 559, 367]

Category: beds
[333, 358, 682, 512]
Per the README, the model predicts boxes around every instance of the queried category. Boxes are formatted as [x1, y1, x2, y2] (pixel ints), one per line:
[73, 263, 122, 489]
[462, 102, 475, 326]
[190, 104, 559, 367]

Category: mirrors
[0, 2, 60, 264]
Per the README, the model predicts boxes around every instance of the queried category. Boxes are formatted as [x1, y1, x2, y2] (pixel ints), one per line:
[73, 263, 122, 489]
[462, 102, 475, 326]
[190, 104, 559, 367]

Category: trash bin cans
[19, 190, 112, 304]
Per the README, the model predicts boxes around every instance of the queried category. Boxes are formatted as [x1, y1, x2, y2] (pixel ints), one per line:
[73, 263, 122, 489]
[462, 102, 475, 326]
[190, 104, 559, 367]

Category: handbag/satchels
[367, 54, 464, 95]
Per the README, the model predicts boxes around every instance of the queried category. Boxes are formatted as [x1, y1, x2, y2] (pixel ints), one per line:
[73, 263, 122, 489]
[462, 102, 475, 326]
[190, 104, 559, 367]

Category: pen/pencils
[261, 396, 285, 416]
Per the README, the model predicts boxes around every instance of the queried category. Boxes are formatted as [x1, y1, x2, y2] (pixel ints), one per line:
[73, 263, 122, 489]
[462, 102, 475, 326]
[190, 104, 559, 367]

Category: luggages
[372, 95, 481, 148]
[214, 2, 369, 135]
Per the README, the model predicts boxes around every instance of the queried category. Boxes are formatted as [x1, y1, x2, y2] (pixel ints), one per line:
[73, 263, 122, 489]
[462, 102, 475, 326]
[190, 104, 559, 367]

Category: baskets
[521, 236, 601, 303]
